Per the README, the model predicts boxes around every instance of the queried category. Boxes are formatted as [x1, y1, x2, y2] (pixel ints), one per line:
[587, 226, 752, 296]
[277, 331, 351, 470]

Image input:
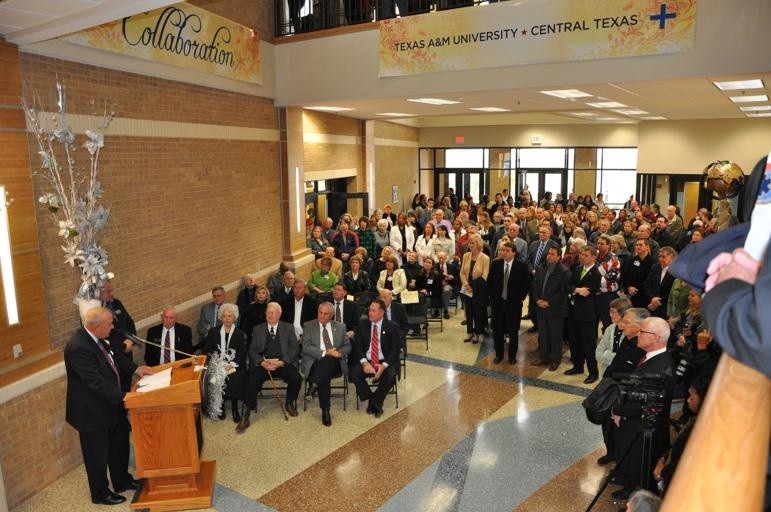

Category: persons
[145, 255, 410, 432]
[80, 280, 137, 392]
[63, 306, 140, 506]
[668, 151, 771, 379]
[304, 184, 716, 384]
[593, 298, 716, 512]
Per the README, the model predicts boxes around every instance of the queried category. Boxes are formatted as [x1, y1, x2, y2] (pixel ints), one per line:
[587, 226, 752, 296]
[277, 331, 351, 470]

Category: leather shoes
[493, 357, 502, 363]
[115, 479, 140, 493]
[322, 409, 332, 426]
[530, 350, 557, 371]
[564, 367, 584, 375]
[461, 321, 478, 344]
[509, 359, 516, 364]
[92, 492, 126, 505]
[218, 405, 249, 432]
[584, 376, 594, 383]
[286, 401, 298, 416]
[521, 313, 529, 319]
[527, 324, 537, 332]
[598, 454, 629, 500]
[367, 400, 384, 417]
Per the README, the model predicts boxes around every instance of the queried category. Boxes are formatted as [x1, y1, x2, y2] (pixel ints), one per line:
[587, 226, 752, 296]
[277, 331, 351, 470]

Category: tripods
[586, 423, 656, 512]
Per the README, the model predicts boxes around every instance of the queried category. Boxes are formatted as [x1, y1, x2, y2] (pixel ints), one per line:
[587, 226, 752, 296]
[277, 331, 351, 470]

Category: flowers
[5, 72, 117, 301]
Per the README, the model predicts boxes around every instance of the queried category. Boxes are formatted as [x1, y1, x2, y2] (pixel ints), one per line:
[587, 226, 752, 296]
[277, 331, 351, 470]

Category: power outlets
[13, 344, 23, 359]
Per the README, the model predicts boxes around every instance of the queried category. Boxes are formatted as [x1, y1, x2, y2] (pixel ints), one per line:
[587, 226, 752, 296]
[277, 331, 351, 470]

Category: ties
[534, 243, 545, 269]
[164, 331, 171, 363]
[371, 324, 379, 370]
[97, 341, 121, 388]
[521, 221, 526, 238]
[322, 324, 333, 350]
[270, 327, 275, 340]
[336, 301, 341, 323]
[501, 264, 508, 300]
[216, 306, 222, 326]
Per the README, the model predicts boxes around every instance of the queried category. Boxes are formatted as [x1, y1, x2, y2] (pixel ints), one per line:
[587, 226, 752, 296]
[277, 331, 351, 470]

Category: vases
[79, 299, 101, 320]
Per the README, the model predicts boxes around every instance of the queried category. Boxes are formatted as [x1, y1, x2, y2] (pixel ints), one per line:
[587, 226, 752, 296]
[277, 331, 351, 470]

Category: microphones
[118, 328, 143, 348]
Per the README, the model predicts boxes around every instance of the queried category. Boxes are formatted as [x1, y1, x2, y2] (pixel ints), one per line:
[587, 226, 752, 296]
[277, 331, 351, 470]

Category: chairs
[254, 292, 464, 413]
